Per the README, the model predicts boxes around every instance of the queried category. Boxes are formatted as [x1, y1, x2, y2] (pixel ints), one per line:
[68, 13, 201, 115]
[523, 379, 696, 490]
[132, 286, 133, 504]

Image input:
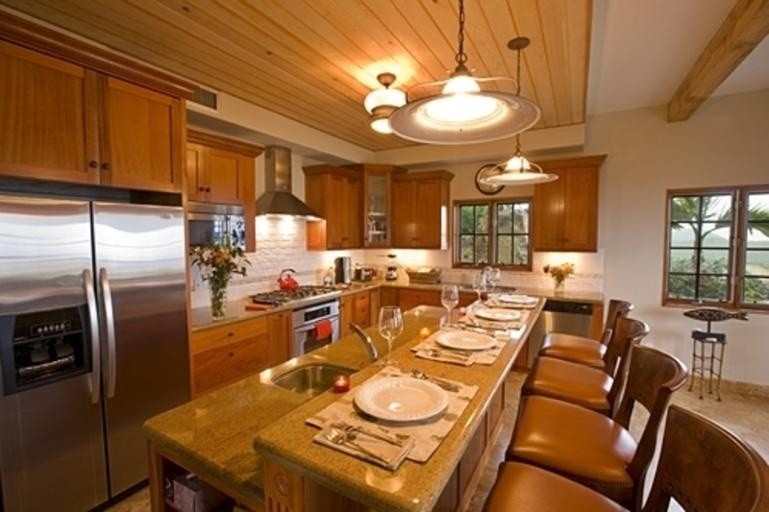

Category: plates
[498, 294, 537, 304]
[435, 330, 499, 352]
[353, 376, 449, 422]
[475, 309, 521, 322]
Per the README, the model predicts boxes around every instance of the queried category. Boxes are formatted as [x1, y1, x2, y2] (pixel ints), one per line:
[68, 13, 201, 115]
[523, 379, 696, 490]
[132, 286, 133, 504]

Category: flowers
[188, 234, 252, 317]
[543, 262, 575, 289]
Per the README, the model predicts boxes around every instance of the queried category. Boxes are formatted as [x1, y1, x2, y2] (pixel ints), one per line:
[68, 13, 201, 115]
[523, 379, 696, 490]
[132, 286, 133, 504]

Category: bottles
[385, 254, 397, 281]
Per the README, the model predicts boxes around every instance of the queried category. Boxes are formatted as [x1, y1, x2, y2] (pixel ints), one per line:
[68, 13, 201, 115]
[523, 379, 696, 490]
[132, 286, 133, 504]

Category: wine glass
[376, 305, 404, 367]
[435, 266, 500, 327]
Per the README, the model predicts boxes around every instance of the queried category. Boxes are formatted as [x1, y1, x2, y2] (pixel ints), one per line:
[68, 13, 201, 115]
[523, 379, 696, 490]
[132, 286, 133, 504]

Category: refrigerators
[0, 191, 194, 512]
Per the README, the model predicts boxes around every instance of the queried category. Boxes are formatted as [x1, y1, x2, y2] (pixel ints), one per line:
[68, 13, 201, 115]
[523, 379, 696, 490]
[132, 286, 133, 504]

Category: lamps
[362, 0, 561, 189]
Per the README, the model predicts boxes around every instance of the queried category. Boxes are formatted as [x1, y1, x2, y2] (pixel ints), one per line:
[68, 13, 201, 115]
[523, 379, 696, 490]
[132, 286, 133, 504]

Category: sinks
[261, 353, 359, 403]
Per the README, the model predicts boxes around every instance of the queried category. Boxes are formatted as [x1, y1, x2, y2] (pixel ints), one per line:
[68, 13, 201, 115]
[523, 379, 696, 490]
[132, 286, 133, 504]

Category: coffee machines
[333, 256, 352, 285]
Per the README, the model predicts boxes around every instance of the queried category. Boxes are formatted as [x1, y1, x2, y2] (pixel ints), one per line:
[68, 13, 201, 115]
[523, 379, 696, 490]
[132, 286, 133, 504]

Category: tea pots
[277, 268, 300, 292]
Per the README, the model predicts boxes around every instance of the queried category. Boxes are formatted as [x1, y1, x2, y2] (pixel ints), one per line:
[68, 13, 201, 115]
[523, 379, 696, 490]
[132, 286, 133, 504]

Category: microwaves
[185, 211, 246, 254]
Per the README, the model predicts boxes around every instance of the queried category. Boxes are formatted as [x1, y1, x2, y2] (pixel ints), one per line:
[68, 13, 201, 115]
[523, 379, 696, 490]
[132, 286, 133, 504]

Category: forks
[420, 346, 471, 365]
[410, 367, 459, 392]
[324, 416, 406, 464]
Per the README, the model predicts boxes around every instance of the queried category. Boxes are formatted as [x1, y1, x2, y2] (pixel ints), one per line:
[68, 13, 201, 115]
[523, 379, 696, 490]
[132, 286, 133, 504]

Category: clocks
[474, 164, 504, 195]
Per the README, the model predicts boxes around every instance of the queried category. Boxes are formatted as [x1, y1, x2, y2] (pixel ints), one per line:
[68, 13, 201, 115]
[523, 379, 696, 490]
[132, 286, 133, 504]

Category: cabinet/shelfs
[189, 307, 293, 401]
[532, 164, 599, 253]
[383, 285, 488, 320]
[337, 288, 381, 336]
[301, 163, 455, 252]
[186, 141, 256, 209]
[1, 35, 191, 194]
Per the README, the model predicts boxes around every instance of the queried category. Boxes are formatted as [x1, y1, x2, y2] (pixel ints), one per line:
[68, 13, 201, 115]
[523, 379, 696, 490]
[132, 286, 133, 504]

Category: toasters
[356, 266, 377, 282]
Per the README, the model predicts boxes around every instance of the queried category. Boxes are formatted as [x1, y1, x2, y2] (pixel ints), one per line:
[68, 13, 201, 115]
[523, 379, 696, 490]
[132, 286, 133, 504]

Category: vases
[209, 281, 227, 320]
[554, 280, 565, 298]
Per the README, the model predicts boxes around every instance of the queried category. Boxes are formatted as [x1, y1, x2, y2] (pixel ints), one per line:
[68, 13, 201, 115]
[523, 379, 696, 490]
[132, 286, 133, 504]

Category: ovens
[292, 300, 340, 357]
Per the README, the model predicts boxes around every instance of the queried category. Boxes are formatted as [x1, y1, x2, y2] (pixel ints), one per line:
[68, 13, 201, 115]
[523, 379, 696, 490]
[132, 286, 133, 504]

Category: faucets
[279, 267, 296, 278]
[348, 320, 379, 363]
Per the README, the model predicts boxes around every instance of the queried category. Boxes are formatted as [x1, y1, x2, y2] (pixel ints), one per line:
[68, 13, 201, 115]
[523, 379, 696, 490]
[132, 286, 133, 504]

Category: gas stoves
[250, 286, 342, 303]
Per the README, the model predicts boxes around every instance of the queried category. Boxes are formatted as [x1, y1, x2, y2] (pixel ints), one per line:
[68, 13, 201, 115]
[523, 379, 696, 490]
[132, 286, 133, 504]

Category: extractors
[252, 145, 323, 223]
